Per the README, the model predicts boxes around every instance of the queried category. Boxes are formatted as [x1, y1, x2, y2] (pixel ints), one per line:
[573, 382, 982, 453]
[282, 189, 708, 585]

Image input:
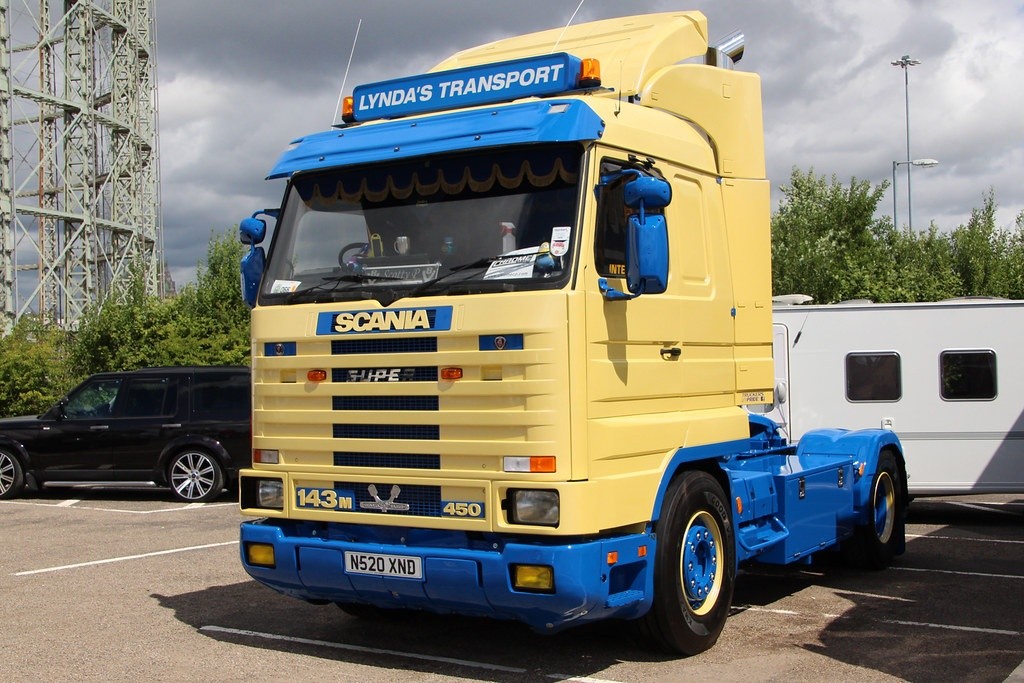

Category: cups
[393, 236, 409, 255]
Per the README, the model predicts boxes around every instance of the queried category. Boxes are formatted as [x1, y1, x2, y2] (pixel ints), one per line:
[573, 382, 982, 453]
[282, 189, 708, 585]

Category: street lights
[890, 54, 922, 236]
[892, 157, 940, 236]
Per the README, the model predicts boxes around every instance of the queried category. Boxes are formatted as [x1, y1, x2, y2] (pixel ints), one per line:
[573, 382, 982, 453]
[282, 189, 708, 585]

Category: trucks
[239, 10, 913, 660]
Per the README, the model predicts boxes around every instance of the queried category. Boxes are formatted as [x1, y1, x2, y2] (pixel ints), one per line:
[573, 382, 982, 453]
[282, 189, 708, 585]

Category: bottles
[344, 259, 366, 274]
[442, 237, 459, 268]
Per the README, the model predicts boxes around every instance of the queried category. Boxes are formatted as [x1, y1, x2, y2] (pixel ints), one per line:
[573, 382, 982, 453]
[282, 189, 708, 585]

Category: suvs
[0, 365, 256, 503]
